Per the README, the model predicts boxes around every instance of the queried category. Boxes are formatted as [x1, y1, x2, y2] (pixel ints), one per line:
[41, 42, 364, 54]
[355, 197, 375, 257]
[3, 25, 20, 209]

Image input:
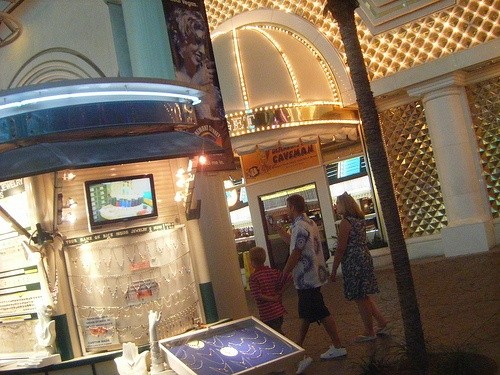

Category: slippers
[376, 326, 391, 334]
[353, 334, 376, 343]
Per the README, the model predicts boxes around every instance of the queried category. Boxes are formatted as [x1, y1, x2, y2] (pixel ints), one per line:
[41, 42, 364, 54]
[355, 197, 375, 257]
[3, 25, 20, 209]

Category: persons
[332, 192, 390, 344]
[282, 194, 347, 375]
[247, 247, 293, 336]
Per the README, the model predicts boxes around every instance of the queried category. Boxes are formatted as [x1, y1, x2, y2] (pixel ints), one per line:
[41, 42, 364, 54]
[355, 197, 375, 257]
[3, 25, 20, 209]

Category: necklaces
[0, 322, 36, 354]
[38, 244, 58, 306]
[67, 231, 200, 352]
[169, 327, 284, 375]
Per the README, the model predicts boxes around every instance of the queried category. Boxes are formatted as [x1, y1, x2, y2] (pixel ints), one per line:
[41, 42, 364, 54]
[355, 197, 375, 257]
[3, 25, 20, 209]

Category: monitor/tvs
[84, 174, 159, 233]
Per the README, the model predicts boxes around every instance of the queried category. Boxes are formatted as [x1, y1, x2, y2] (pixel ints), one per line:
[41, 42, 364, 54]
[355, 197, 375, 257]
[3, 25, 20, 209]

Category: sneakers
[319, 346, 347, 359]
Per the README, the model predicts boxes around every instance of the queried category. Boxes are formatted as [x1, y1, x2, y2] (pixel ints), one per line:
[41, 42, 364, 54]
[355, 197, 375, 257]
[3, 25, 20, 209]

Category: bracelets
[275, 227, 282, 231]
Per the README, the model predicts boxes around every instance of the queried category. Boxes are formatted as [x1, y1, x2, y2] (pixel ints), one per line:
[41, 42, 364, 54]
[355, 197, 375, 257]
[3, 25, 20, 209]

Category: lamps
[62, 197, 78, 210]
[63, 211, 77, 224]
[175, 168, 192, 202]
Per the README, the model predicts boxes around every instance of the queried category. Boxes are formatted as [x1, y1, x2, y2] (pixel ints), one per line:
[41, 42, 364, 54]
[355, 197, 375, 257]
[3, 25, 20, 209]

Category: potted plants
[321, 228, 392, 276]
